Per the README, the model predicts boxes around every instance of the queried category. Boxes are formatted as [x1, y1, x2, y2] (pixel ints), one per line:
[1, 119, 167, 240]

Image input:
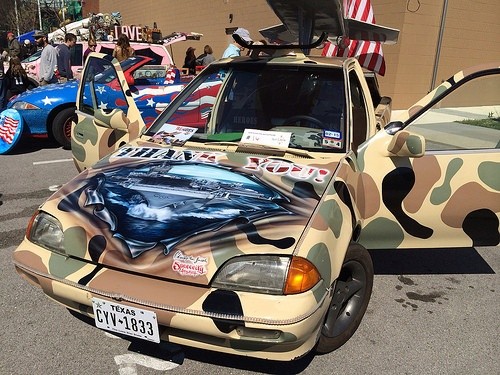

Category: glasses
[35, 37, 41, 40]
[25, 44, 29, 45]
[89, 44, 97, 47]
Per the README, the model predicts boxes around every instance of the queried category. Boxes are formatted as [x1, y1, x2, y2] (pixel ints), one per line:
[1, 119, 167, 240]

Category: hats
[25, 40, 30, 44]
[8, 33, 12, 37]
[31, 30, 48, 37]
[188, 47, 196, 53]
[235, 28, 253, 41]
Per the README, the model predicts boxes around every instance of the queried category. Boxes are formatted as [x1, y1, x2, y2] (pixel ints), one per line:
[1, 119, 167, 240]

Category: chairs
[164, 65, 180, 84]
[219, 73, 366, 146]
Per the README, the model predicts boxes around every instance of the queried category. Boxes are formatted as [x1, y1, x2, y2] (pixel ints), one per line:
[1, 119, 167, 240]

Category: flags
[323, 0, 386, 77]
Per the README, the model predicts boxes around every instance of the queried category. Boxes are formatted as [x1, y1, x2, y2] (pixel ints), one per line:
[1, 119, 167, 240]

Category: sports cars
[9, 56, 227, 150]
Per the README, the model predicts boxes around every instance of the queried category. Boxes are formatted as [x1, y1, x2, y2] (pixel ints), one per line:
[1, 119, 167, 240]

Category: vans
[4, 32, 204, 83]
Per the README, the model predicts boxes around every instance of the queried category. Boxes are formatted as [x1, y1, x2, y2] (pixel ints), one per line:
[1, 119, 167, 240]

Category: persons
[183, 47, 196, 74]
[83, 39, 98, 64]
[195, 44, 214, 66]
[19, 38, 36, 57]
[4, 58, 28, 97]
[5, 32, 20, 59]
[33, 30, 58, 85]
[53, 34, 77, 81]
[221, 28, 251, 58]
[112, 34, 135, 65]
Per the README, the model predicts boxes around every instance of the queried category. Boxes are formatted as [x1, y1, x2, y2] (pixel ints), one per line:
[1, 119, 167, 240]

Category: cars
[16, 0, 500, 363]
